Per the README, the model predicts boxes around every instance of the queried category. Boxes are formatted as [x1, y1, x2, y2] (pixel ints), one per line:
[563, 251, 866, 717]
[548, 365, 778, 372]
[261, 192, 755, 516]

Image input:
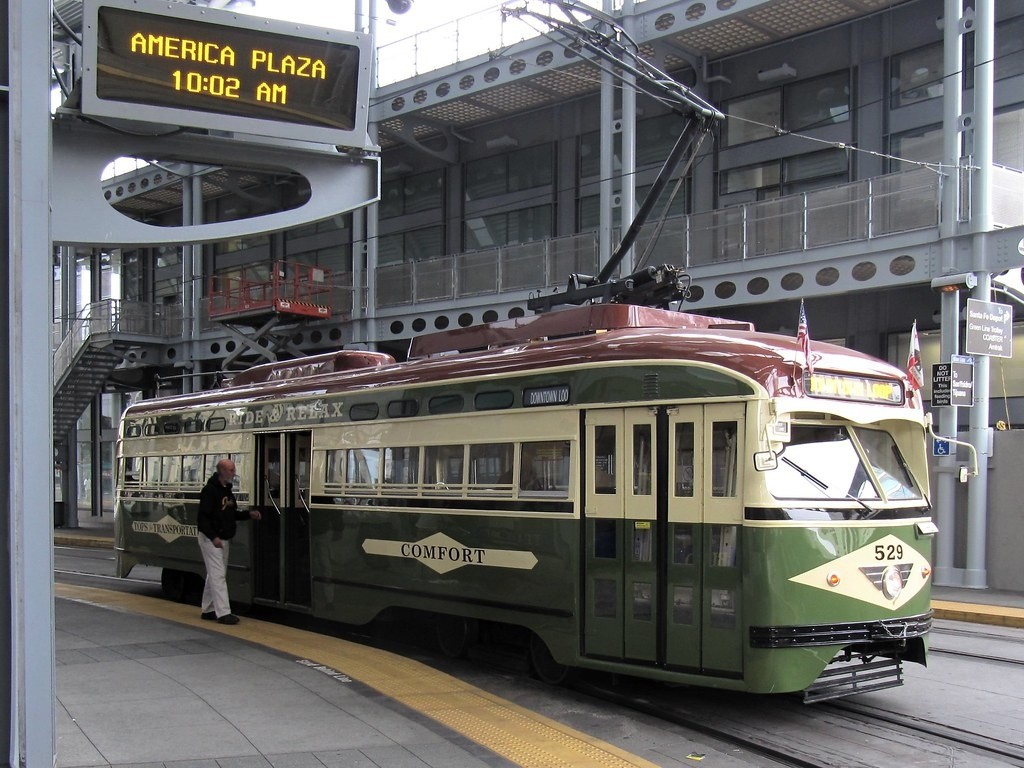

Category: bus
[113, 305, 934, 713]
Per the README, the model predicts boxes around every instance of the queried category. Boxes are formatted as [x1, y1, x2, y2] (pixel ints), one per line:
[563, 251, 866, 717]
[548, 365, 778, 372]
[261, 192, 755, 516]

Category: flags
[908, 323, 924, 391]
[798, 301, 814, 374]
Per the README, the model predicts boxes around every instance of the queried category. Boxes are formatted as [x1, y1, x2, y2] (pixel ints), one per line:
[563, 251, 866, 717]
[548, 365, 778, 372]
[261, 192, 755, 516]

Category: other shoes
[200, 610, 218, 620]
[216, 614, 240, 625]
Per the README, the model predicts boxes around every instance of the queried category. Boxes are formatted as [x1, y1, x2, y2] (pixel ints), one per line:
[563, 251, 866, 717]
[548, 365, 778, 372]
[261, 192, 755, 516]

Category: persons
[496, 445, 543, 491]
[196, 459, 261, 624]
